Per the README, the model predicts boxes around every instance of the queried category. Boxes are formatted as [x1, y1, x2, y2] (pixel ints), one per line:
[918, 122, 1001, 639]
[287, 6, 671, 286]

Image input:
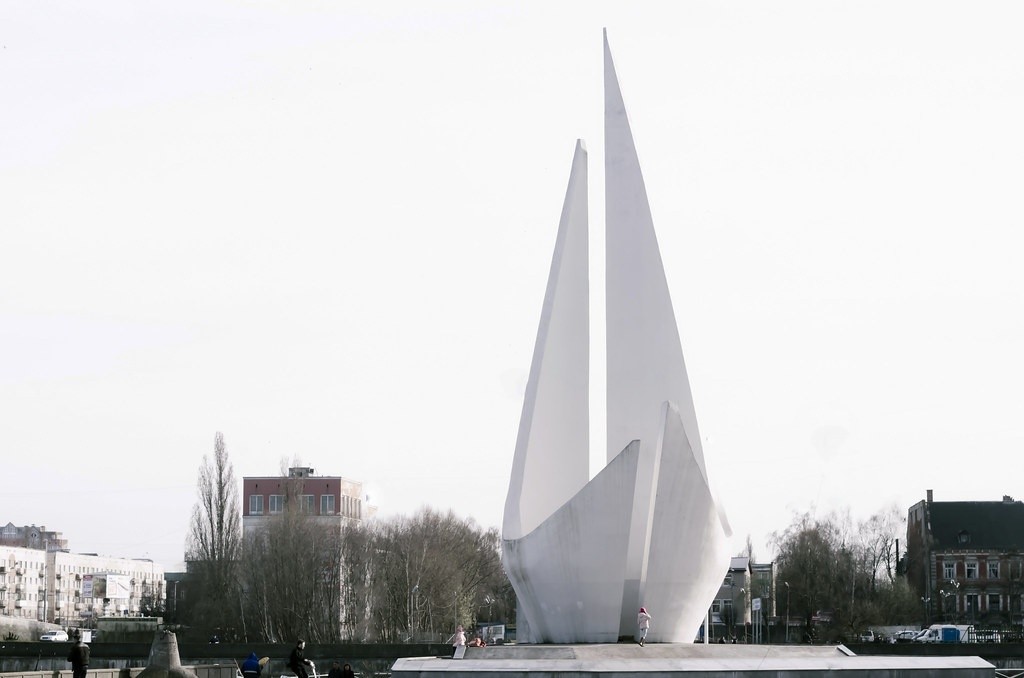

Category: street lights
[174, 579, 180, 609]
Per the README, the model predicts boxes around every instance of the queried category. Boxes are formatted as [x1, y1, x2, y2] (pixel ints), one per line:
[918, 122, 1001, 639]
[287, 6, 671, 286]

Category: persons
[452, 625, 486, 647]
[66, 626, 90, 678]
[718, 634, 738, 644]
[240, 639, 355, 678]
[637, 607, 651, 647]
[208, 636, 220, 644]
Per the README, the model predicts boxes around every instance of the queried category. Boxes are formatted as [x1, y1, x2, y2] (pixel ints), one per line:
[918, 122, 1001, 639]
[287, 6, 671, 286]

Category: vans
[859, 630, 875, 643]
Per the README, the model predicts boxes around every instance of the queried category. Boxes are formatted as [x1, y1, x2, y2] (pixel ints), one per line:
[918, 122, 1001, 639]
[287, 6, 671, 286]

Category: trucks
[911, 623, 978, 644]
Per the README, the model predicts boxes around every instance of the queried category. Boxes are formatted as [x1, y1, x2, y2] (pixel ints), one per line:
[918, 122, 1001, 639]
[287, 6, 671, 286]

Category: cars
[40, 630, 69, 642]
[974, 630, 1001, 643]
